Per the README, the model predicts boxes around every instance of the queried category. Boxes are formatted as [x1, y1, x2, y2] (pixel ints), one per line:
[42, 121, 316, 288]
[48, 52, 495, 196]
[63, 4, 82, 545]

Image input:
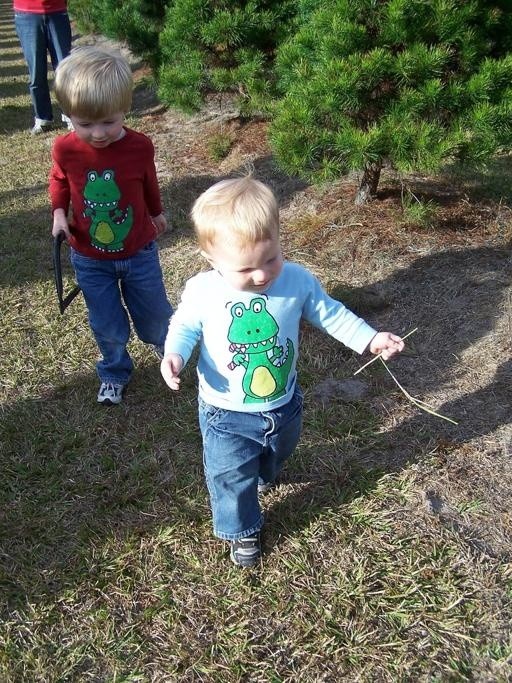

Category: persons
[11, 0, 71, 136]
[47, 47, 173, 406]
[159, 177, 405, 565]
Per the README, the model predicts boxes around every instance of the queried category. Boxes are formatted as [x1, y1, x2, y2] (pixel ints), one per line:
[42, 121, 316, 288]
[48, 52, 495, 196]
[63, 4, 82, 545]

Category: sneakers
[30, 116, 54, 135]
[95, 382, 128, 406]
[230, 529, 262, 568]
[153, 350, 165, 361]
[257, 480, 272, 495]
[66, 121, 74, 131]
